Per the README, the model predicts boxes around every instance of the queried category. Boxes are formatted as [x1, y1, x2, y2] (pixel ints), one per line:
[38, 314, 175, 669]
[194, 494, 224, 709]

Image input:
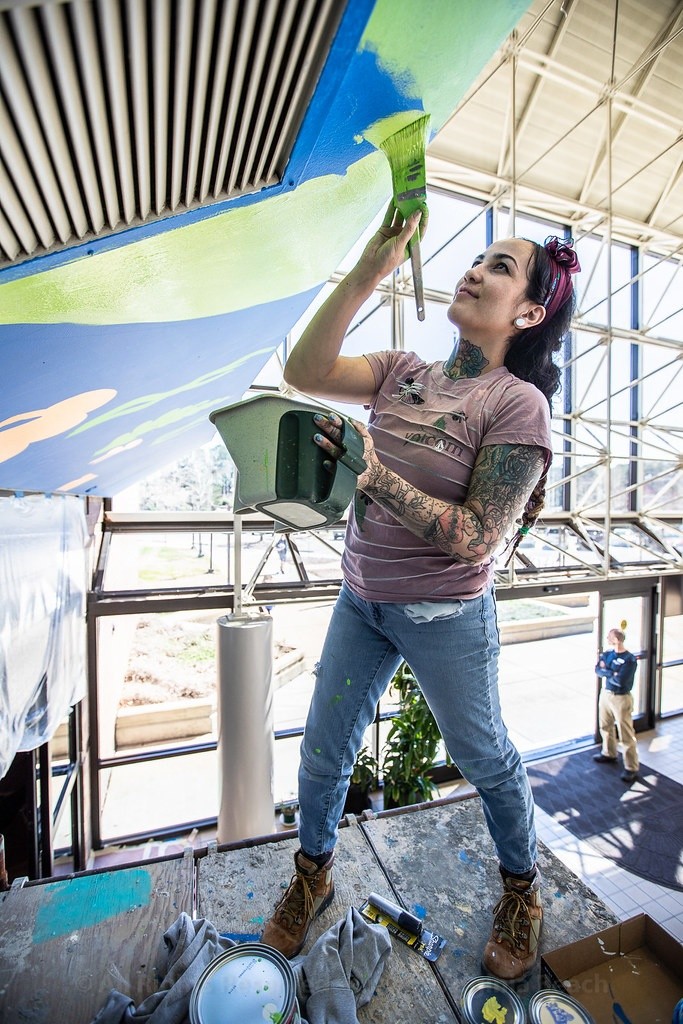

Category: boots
[261, 850, 335, 961]
[483, 868, 545, 988]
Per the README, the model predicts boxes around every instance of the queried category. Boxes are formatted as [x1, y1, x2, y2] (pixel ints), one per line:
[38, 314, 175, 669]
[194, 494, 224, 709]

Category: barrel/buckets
[189, 942, 303, 1024]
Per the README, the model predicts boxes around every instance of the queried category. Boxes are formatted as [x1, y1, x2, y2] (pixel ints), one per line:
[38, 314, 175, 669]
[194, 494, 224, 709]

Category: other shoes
[592, 753, 620, 762]
[620, 769, 640, 782]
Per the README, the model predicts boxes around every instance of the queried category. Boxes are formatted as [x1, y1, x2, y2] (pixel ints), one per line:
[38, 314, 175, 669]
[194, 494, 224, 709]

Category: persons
[593, 629, 640, 782]
[263, 198, 578, 982]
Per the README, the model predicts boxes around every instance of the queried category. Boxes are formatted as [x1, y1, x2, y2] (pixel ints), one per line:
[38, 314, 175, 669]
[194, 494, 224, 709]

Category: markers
[367, 892, 422, 935]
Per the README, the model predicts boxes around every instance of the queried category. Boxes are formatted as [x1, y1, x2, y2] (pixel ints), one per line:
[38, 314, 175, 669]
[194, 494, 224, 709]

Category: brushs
[378, 112, 433, 323]
